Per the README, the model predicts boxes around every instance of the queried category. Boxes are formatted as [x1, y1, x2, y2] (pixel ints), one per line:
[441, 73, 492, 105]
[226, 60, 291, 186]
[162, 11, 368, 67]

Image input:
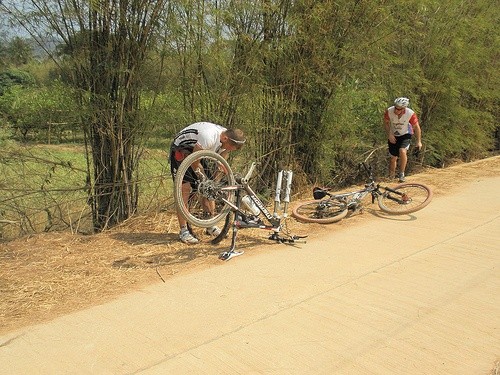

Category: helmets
[394, 96, 410, 108]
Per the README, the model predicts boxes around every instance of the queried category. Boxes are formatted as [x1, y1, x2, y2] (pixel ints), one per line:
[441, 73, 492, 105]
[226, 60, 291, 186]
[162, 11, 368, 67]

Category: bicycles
[174, 151, 308, 262]
[291, 159, 434, 224]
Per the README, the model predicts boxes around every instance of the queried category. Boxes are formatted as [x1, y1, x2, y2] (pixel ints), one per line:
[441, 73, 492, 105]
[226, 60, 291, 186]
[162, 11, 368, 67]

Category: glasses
[395, 107, 403, 111]
[229, 137, 246, 146]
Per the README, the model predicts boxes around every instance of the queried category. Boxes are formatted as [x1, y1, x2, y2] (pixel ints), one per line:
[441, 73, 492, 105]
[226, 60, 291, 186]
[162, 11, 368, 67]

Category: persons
[384, 97, 422, 183]
[170, 122, 245, 244]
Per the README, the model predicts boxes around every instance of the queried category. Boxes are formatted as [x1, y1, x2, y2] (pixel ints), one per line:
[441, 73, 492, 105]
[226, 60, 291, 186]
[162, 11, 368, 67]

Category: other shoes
[179, 229, 198, 244]
[398, 172, 406, 183]
[205, 225, 229, 238]
